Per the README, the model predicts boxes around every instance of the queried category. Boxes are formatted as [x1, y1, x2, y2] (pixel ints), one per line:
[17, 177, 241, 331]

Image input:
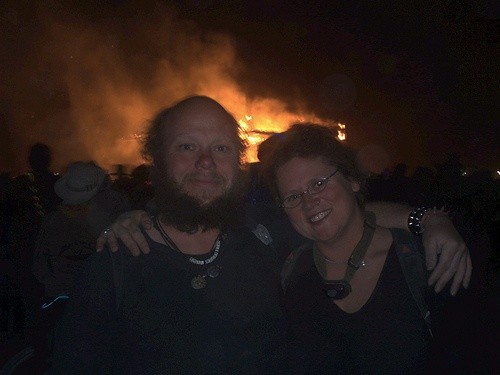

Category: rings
[103, 227, 112, 238]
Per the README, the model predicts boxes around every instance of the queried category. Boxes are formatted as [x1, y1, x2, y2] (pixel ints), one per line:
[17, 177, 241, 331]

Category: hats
[53, 161, 104, 205]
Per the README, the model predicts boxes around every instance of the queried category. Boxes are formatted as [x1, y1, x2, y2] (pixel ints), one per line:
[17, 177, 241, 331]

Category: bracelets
[408, 205, 427, 234]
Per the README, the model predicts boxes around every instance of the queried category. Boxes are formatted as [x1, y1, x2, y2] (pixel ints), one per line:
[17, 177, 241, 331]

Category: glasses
[276, 169, 342, 211]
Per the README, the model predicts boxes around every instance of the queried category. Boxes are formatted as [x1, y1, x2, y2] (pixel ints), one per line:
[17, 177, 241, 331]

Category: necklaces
[149, 212, 225, 291]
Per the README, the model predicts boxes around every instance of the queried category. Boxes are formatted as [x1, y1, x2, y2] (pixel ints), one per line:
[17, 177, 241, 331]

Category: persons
[0, 143, 155, 342]
[97, 124, 442, 375]
[46, 97, 470, 375]
